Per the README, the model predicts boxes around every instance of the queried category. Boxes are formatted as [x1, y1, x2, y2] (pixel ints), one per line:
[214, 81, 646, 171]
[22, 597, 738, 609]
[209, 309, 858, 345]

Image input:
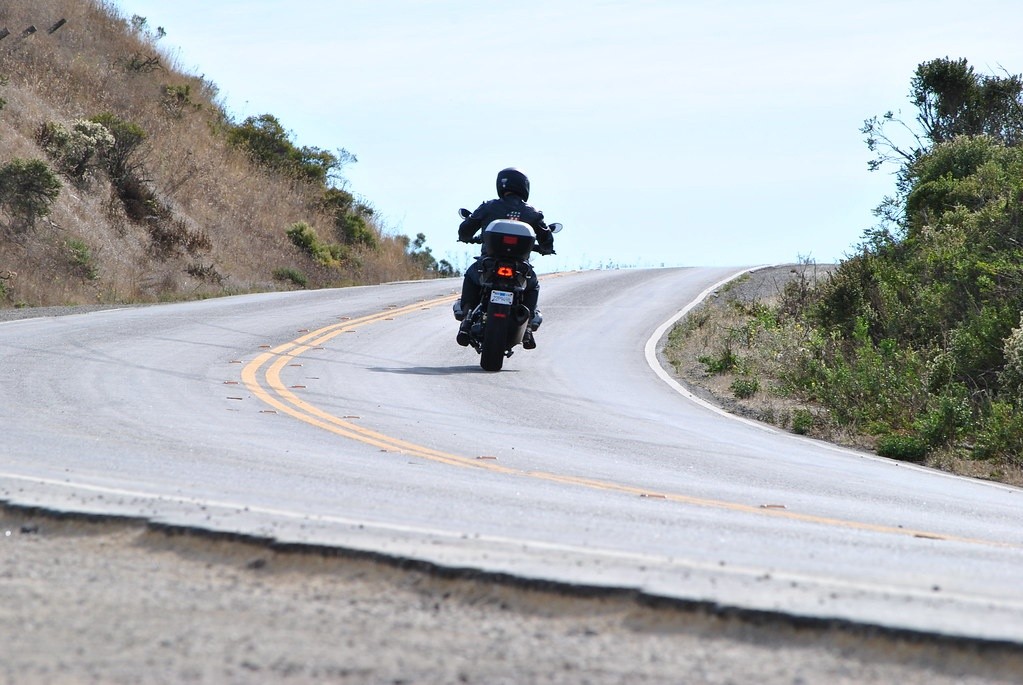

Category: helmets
[496, 167, 530, 203]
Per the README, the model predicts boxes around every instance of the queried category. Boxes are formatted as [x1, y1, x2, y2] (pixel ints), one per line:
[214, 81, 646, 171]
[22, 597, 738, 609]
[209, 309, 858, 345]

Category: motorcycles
[456, 208, 562, 371]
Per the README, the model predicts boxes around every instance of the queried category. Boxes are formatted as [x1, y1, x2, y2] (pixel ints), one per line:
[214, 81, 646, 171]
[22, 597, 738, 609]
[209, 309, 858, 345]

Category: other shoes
[455, 320, 470, 347]
[523, 329, 536, 350]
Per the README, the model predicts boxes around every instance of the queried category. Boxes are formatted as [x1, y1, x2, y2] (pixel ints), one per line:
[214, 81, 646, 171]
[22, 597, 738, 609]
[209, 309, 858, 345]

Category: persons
[458, 168, 554, 349]
